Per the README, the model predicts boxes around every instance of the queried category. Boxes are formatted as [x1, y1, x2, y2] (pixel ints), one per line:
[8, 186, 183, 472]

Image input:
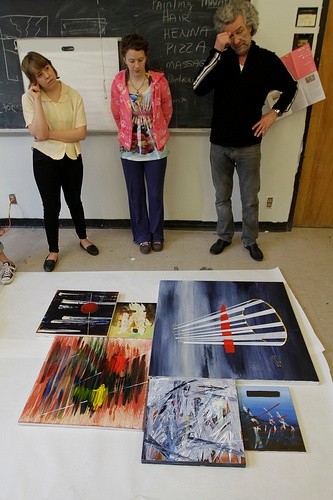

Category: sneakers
[0, 262, 16, 284]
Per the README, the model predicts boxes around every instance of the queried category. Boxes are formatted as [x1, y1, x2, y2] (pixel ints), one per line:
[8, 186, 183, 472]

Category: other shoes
[140, 240, 151, 254]
[43, 254, 58, 272]
[152, 241, 163, 251]
[246, 243, 263, 261]
[210, 239, 231, 254]
[80, 240, 99, 256]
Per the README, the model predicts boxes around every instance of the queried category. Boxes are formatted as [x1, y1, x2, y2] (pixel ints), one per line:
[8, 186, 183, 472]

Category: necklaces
[130, 75, 146, 95]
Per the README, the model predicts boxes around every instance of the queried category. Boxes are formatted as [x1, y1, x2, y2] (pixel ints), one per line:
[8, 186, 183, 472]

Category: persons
[0, 242, 17, 285]
[110, 34, 172, 254]
[21, 51, 99, 273]
[190, 0, 297, 262]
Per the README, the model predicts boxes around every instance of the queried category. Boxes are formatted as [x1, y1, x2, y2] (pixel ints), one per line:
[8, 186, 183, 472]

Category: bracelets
[274, 109, 281, 114]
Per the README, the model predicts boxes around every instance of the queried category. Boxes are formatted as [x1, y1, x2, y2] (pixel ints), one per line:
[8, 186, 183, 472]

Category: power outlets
[266, 196, 273, 207]
[9, 193, 16, 204]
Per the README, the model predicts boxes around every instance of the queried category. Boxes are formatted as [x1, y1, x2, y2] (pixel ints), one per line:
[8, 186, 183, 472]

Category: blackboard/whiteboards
[0, 0, 253, 138]
